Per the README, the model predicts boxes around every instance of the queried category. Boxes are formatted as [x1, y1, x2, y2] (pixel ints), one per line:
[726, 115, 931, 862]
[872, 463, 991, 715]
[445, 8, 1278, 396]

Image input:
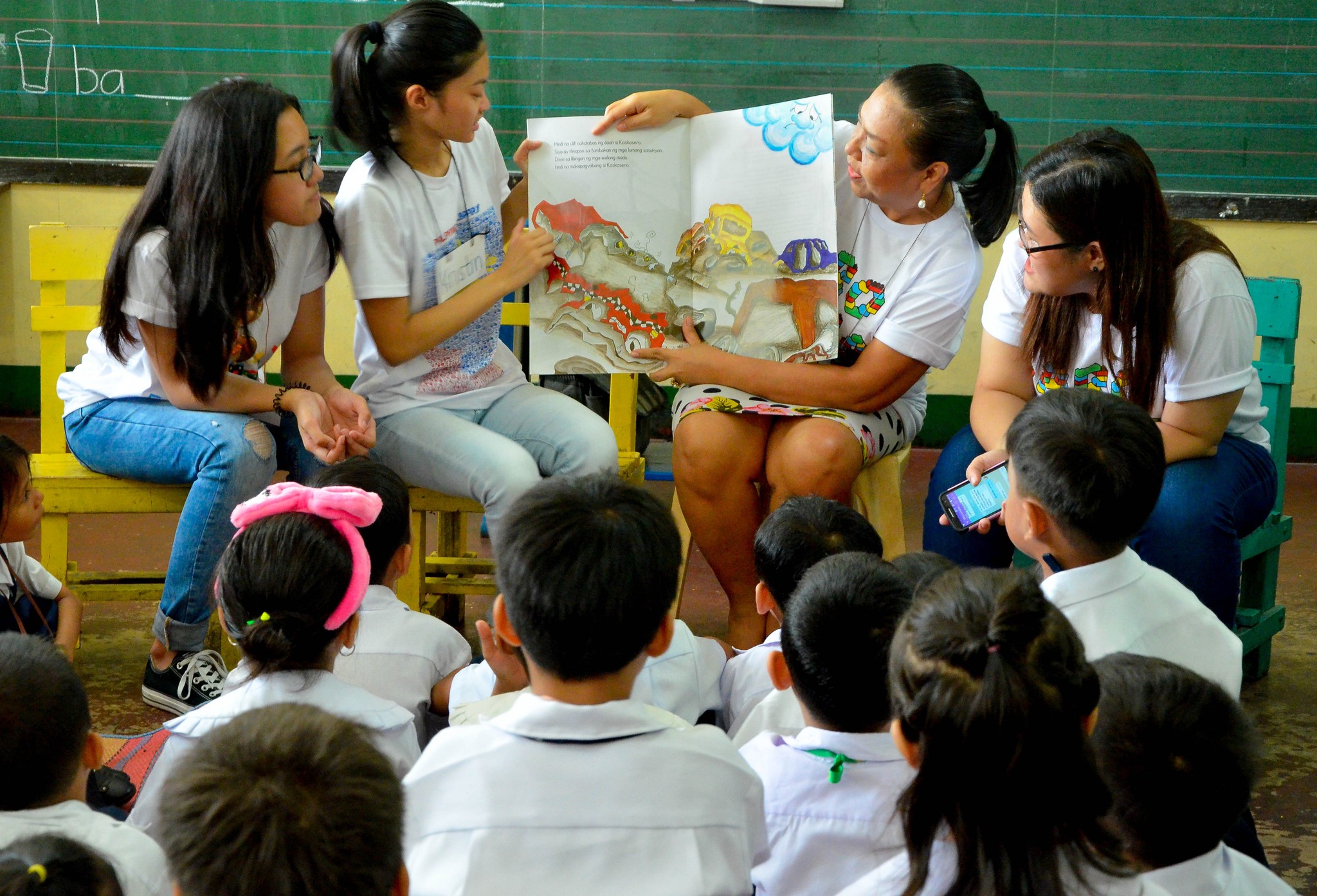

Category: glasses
[263, 134, 324, 183]
[1014, 201, 1097, 257]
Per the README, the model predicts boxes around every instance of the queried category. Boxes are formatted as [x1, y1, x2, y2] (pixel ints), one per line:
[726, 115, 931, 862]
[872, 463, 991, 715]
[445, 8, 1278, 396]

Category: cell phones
[940, 459, 1010, 532]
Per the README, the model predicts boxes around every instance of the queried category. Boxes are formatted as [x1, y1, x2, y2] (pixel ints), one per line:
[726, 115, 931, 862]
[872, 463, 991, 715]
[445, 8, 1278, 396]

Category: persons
[218, 455, 477, 749]
[1004, 389, 1245, 705]
[1087, 651, 1301, 896]
[160, 703, 410, 896]
[919, 127, 1279, 632]
[448, 620, 739, 733]
[0, 629, 174, 896]
[591, 62, 1019, 653]
[120, 480, 422, 846]
[56, 83, 378, 715]
[826, 567, 1173, 896]
[401, 473, 771, 896]
[0, 832, 124, 896]
[884, 550, 955, 593]
[718, 494, 885, 726]
[329, 1, 622, 564]
[740, 550, 920, 896]
[0, 434, 82, 667]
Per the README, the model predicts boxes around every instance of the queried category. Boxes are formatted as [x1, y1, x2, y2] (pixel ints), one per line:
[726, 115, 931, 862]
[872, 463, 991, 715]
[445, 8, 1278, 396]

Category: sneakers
[137, 640, 229, 717]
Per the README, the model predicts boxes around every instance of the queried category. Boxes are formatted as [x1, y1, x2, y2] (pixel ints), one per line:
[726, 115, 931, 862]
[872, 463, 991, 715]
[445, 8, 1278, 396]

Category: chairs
[26, 219, 288, 674]
[1012, 272, 1304, 683]
[394, 300, 650, 623]
[657, 441, 907, 619]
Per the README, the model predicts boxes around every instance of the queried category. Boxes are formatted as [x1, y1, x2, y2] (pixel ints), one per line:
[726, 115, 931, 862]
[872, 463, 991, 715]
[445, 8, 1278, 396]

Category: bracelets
[272, 381, 315, 419]
